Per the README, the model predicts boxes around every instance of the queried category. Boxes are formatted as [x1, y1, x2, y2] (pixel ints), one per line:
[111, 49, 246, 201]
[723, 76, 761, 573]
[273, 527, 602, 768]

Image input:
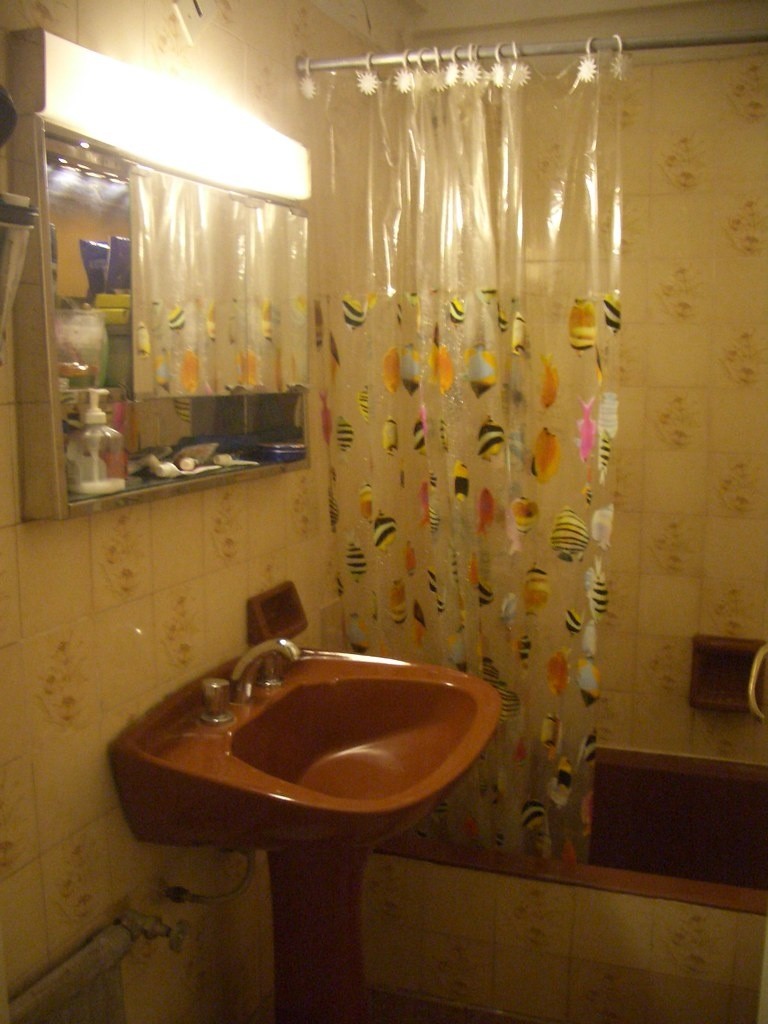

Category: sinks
[217, 668, 503, 850]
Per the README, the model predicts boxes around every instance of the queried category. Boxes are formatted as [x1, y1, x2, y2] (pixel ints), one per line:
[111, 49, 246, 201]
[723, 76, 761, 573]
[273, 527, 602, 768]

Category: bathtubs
[361, 729, 768, 1024]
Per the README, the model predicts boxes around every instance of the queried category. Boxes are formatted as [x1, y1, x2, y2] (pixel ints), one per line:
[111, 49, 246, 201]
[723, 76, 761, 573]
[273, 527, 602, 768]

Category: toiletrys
[64, 385, 306, 496]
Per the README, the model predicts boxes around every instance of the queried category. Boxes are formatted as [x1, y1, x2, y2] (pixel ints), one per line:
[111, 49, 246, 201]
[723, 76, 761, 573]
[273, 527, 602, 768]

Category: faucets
[229, 636, 300, 706]
